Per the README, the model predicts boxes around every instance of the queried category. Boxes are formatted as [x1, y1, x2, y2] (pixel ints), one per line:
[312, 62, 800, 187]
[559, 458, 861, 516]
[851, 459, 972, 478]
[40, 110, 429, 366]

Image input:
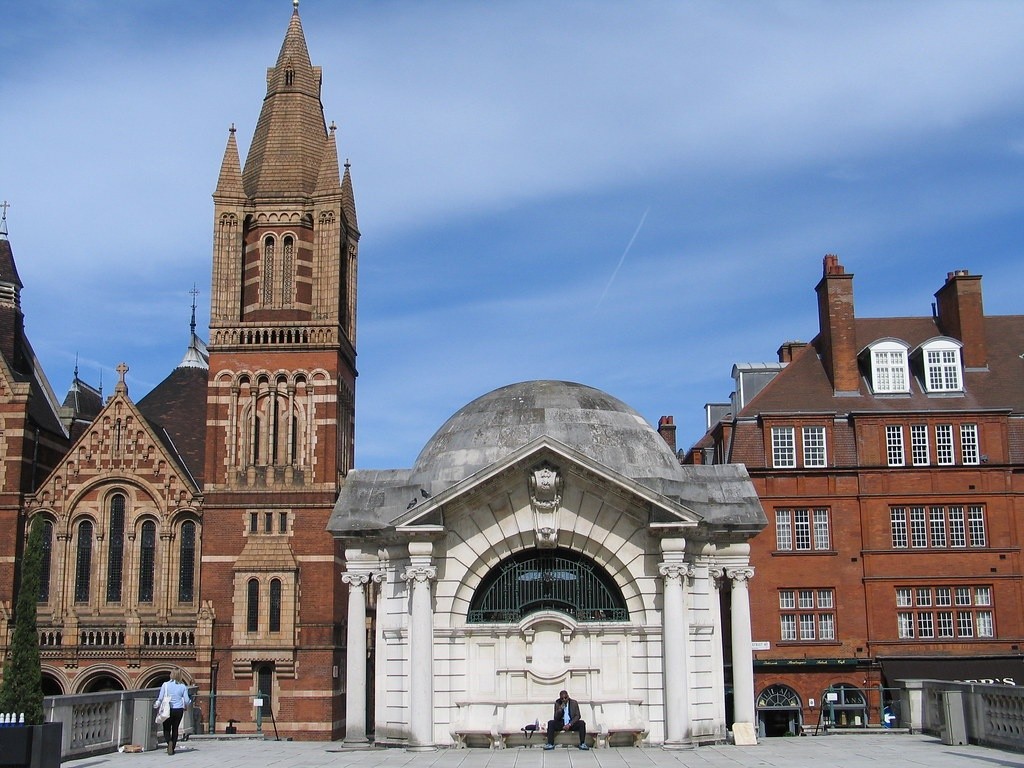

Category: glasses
[560, 695, 568, 700]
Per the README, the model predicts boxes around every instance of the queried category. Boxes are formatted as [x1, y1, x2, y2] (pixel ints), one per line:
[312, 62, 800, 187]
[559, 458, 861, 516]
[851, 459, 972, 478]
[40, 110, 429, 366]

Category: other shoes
[167, 741, 173, 755]
[543, 743, 555, 750]
[578, 743, 589, 750]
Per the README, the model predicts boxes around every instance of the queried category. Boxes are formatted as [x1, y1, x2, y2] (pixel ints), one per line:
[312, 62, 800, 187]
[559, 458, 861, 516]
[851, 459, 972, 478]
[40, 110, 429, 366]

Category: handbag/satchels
[525, 725, 536, 740]
[154, 682, 171, 724]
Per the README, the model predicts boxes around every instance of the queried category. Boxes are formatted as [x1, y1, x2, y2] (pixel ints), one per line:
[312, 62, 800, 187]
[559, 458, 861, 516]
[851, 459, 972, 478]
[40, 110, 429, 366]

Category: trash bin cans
[936, 690, 968, 745]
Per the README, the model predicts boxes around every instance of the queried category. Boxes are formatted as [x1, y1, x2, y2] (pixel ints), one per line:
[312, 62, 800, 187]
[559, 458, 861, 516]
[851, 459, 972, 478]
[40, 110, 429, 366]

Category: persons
[153, 667, 191, 755]
[543, 690, 589, 750]
